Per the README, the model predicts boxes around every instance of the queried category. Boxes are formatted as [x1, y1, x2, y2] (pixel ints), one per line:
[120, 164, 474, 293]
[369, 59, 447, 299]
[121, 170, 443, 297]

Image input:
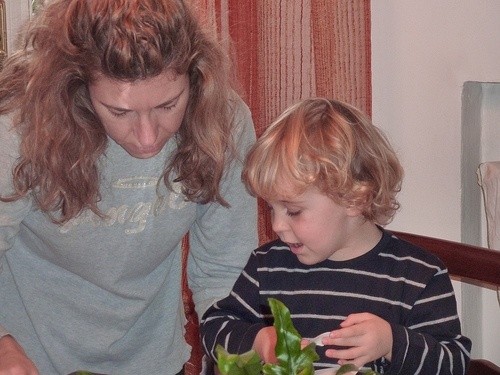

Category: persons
[199, 96, 474, 374]
[0, 1, 261, 375]
